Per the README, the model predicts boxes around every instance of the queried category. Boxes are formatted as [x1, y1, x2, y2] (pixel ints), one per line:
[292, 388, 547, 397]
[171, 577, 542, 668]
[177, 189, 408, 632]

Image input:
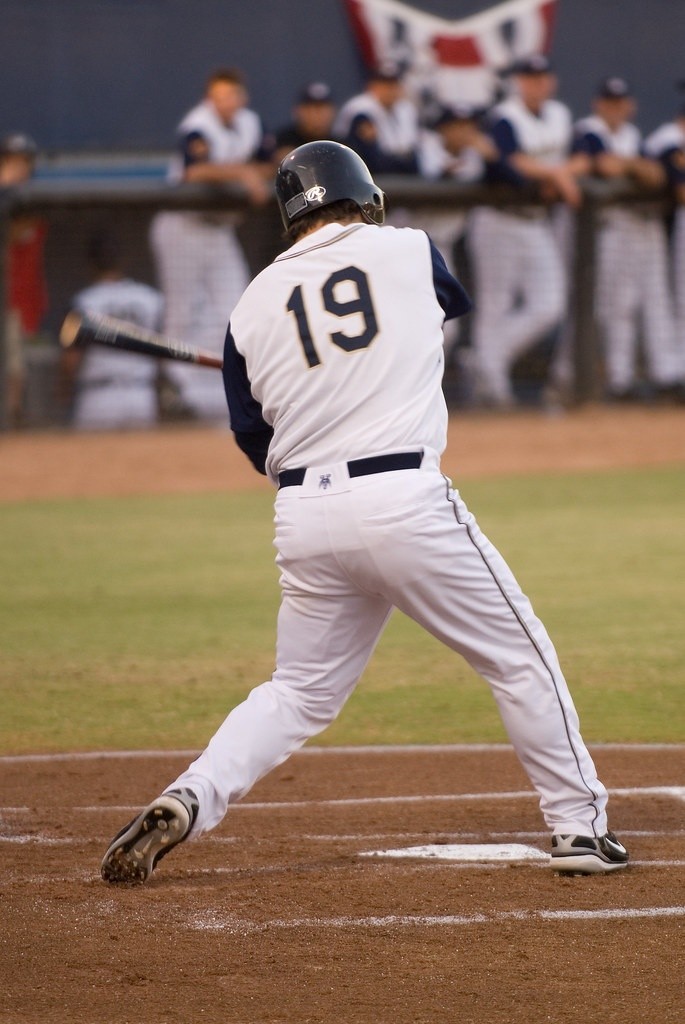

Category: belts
[278, 452, 424, 491]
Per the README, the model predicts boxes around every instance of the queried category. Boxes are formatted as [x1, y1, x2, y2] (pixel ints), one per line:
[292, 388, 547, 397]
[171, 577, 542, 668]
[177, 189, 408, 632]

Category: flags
[351, 0, 558, 86]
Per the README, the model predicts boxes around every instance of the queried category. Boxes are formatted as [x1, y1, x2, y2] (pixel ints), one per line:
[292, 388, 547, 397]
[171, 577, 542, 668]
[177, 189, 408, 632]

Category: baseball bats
[56, 307, 224, 370]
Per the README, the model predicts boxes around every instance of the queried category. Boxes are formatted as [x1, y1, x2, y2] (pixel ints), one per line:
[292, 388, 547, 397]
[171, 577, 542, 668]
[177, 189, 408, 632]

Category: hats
[370, 62, 400, 80]
[516, 56, 552, 74]
[297, 75, 333, 105]
[597, 77, 629, 99]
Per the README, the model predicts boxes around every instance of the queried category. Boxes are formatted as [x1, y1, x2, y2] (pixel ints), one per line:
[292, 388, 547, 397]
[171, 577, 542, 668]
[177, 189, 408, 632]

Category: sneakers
[550, 828, 630, 877]
[100, 787, 199, 888]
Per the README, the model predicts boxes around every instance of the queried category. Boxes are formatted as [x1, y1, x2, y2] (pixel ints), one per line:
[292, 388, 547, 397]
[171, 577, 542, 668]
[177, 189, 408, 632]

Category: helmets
[274, 140, 390, 231]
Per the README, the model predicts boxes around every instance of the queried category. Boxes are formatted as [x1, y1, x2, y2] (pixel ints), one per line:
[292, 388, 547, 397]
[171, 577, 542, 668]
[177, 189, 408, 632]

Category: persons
[99, 138, 629, 887]
[0, 53, 684, 435]
